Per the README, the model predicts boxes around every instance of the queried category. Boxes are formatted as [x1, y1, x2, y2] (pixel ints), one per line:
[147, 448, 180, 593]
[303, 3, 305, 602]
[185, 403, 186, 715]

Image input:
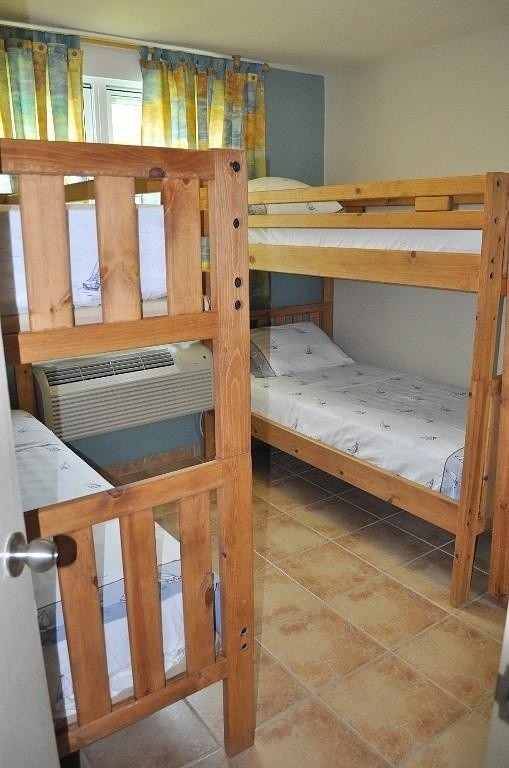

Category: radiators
[30, 341, 213, 444]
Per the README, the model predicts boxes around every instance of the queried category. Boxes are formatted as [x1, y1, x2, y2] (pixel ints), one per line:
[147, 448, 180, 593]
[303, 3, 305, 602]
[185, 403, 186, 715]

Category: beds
[4, 141, 257, 758]
[206, 171, 509, 607]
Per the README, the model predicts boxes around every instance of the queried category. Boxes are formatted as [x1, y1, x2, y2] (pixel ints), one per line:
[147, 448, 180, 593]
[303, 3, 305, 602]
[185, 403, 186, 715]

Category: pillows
[248, 176, 343, 215]
[249, 321, 354, 378]
